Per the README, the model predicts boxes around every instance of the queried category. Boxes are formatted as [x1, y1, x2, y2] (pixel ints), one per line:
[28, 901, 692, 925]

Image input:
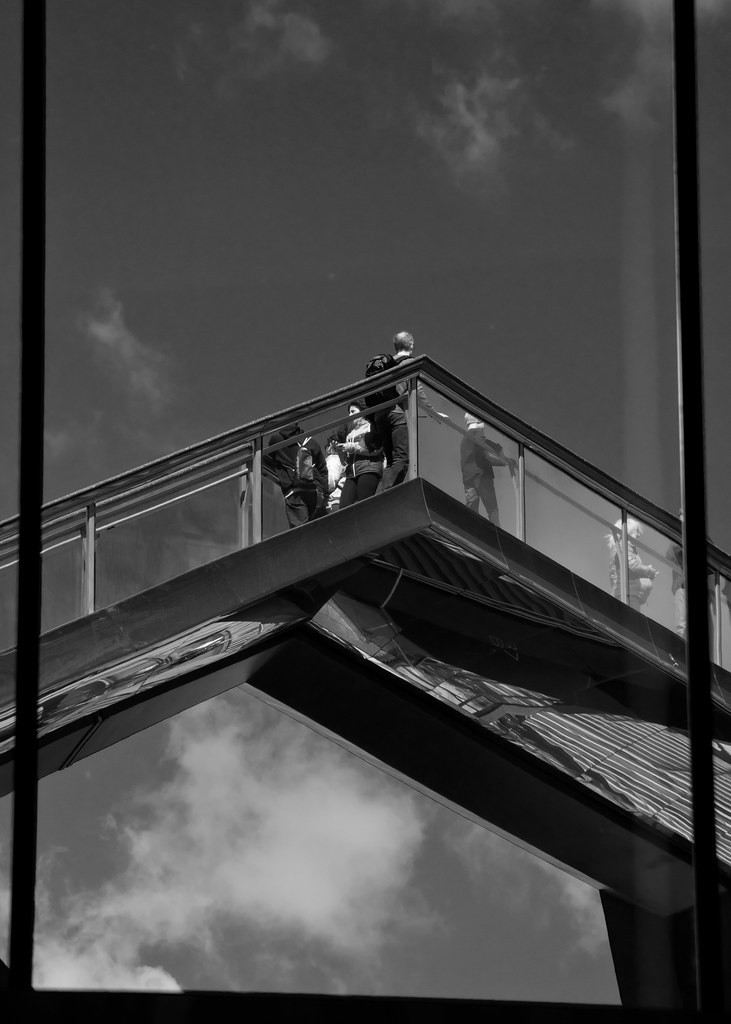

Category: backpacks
[294, 437, 315, 481]
[365, 354, 415, 408]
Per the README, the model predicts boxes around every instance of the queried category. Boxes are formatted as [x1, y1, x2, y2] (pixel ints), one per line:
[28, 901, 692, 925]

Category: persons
[262, 422, 331, 531]
[330, 397, 385, 511]
[322, 430, 348, 514]
[665, 506, 722, 662]
[374, 331, 448, 493]
[459, 413, 517, 530]
[603, 517, 658, 612]
